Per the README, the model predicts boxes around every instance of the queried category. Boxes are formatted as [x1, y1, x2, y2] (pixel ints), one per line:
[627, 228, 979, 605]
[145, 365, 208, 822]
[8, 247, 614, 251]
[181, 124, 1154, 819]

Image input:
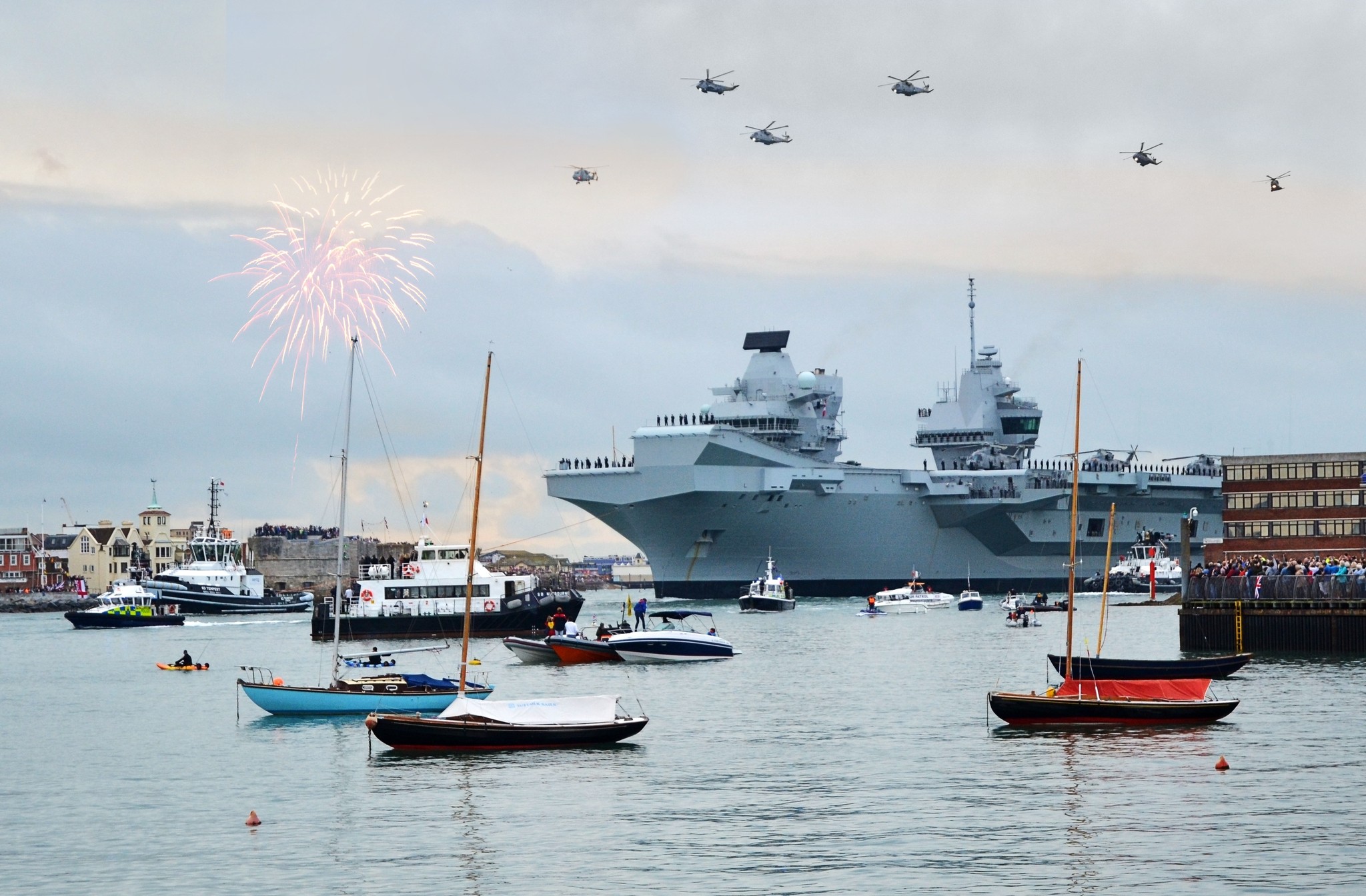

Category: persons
[359, 647, 382, 665]
[256, 522, 340, 540]
[349, 535, 410, 581]
[174, 650, 193, 667]
[6, 585, 77, 593]
[547, 408, 1366, 639]
[330, 585, 354, 612]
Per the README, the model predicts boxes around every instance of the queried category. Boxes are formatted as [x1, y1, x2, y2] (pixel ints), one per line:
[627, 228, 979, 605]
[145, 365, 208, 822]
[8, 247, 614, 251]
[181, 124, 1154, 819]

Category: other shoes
[643, 630, 648, 632]
[635, 629, 637, 632]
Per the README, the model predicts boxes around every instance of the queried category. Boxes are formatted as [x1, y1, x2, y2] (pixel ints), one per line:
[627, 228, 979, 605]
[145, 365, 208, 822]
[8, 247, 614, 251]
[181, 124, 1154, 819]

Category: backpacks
[1223, 567, 1228, 575]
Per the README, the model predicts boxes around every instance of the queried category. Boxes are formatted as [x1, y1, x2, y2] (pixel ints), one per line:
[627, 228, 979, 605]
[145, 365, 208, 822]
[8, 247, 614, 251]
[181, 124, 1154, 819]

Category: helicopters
[1054, 444, 1153, 472]
[1253, 171, 1291, 192]
[740, 121, 793, 147]
[565, 164, 603, 184]
[941, 440, 1042, 471]
[877, 69, 934, 97]
[680, 69, 740, 96]
[1162, 454, 1223, 477]
[1120, 142, 1163, 167]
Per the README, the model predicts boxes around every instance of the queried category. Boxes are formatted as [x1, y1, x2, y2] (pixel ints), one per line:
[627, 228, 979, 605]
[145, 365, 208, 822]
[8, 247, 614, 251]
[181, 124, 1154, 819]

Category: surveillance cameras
[1193, 510, 1199, 517]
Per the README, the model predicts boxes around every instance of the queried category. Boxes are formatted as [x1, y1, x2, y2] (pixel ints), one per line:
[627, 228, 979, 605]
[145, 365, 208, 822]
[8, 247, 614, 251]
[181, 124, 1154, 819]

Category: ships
[539, 276, 1226, 601]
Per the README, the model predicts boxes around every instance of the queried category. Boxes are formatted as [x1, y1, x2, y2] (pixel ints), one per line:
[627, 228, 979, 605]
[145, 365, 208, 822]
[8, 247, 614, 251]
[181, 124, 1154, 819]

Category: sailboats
[1046, 502, 1256, 680]
[365, 349, 652, 755]
[986, 358, 1241, 733]
[237, 335, 497, 718]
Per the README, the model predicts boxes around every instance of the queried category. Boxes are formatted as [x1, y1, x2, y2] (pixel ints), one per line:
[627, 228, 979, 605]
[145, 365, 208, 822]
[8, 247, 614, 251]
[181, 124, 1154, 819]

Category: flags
[593, 614, 597, 621]
[822, 405, 826, 416]
[220, 482, 224, 485]
[44, 551, 88, 599]
[422, 511, 428, 526]
[626, 595, 632, 617]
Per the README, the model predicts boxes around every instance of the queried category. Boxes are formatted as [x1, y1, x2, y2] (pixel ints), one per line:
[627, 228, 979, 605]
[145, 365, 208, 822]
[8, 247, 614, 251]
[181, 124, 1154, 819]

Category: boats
[957, 559, 984, 611]
[330, 653, 408, 693]
[156, 661, 210, 671]
[856, 603, 888, 617]
[64, 578, 185, 630]
[738, 546, 795, 612]
[999, 592, 1077, 628]
[874, 564, 955, 615]
[311, 501, 624, 666]
[608, 609, 743, 665]
[137, 476, 315, 617]
[1084, 531, 1182, 593]
